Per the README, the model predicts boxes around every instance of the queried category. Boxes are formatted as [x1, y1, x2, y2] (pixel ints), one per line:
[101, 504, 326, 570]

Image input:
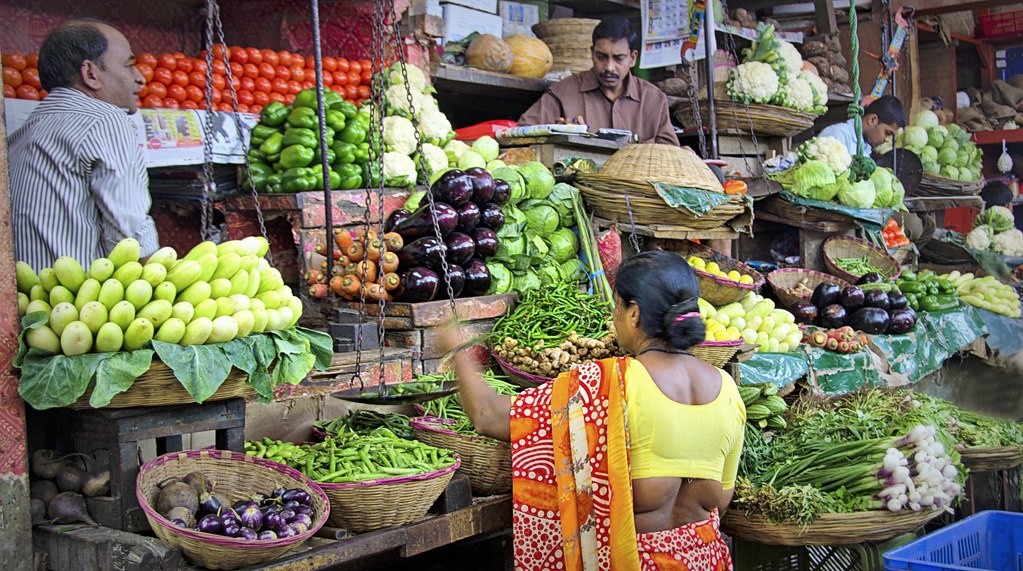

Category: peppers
[243, 87, 382, 193]
[899, 272, 961, 312]
[488, 278, 616, 355]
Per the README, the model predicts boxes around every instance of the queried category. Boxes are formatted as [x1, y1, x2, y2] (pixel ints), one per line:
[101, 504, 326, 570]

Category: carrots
[303, 228, 403, 302]
[802, 325, 868, 354]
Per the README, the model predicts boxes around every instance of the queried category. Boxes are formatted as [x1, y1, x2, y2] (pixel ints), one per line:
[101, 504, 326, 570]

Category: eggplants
[383, 168, 511, 305]
[788, 273, 915, 335]
[169, 479, 315, 550]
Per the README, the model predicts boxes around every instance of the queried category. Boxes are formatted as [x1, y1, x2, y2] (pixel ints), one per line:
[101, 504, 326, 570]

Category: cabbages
[403, 160, 587, 297]
[790, 160, 905, 208]
[874, 108, 982, 182]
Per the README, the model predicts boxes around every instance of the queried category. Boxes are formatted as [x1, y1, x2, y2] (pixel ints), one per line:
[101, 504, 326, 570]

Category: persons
[518, 17, 682, 149]
[435, 250, 746, 570]
[980, 180, 1014, 213]
[817, 95, 906, 158]
[4, 20, 162, 270]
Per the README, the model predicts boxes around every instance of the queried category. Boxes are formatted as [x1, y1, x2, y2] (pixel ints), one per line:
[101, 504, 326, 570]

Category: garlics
[758, 425, 962, 515]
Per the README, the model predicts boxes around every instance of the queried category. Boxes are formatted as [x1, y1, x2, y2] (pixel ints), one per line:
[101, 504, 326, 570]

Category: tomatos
[1, 43, 373, 116]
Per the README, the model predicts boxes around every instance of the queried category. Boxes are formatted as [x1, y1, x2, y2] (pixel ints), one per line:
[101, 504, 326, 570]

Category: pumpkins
[465, 33, 552, 79]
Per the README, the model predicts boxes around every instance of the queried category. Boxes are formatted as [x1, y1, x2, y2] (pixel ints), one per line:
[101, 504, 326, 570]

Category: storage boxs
[534, 16, 604, 79]
[672, 96, 819, 139]
[136, 448, 332, 570]
[303, 452, 466, 532]
[409, 412, 518, 494]
[652, 239, 768, 307]
[576, 169, 751, 232]
[819, 232, 907, 285]
[766, 261, 849, 307]
[717, 504, 949, 548]
[879, 509, 1023, 569]
[689, 339, 745, 372]
[37, 353, 254, 411]
[599, 142, 727, 194]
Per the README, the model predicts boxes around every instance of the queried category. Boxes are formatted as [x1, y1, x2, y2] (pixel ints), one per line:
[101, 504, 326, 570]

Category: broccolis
[965, 206, 1021, 258]
[360, 61, 505, 188]
[725, 24, 851, 176]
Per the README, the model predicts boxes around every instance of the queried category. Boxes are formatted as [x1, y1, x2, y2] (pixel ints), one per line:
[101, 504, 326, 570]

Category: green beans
[243, 423, 456, 483]
